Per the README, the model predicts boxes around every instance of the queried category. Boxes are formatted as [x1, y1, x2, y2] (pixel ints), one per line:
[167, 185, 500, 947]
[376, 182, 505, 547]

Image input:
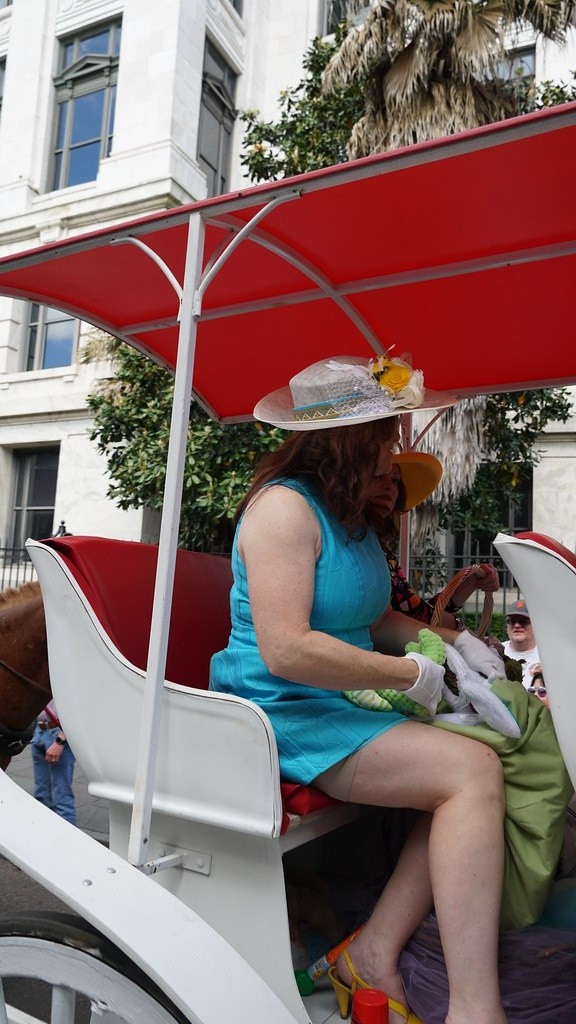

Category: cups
[351, 989, 389, 1024]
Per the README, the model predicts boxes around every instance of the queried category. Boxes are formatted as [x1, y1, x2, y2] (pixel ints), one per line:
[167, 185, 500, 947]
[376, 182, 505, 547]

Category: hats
[382, 440, 445, 516]
[253, 352, 458, 430]
[504, 600, 531, 617]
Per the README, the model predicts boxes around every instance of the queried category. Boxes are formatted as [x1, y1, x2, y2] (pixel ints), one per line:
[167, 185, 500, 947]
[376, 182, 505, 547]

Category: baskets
[430, 560, 523, 688]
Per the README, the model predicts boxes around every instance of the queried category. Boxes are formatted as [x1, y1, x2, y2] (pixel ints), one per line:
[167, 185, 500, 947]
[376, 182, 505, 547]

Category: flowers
[324, 344, 426, 408]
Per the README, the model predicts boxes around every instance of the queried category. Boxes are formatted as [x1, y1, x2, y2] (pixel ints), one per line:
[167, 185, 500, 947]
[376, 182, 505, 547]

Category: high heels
[328, 947, 424, 1024]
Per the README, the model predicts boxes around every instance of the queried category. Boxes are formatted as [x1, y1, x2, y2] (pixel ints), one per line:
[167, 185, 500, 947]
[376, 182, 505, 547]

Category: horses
[1, 533, 73, 774]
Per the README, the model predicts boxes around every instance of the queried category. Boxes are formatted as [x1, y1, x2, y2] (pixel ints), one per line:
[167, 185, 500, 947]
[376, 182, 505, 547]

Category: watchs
[56, 737, 66, 745]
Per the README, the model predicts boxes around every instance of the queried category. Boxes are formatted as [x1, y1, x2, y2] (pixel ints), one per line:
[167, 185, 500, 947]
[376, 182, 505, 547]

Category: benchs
[26, 535, 359, 839]
[493, 530, 576, 790]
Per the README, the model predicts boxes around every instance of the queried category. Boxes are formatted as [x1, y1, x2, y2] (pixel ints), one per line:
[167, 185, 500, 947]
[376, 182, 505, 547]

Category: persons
[527, 663, 551, 712]
[206, 349, 521, 1024]
[503, 600, 540, 689]
[32, 699, 76, 829]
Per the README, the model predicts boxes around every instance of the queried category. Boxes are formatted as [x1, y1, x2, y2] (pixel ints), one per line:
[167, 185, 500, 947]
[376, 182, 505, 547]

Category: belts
[35, 722, 58, 731]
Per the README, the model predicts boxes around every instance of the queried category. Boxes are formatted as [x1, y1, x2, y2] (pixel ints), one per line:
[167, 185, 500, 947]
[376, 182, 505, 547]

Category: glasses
[507, 618, 530, 626]
[527, 686, 548, 700]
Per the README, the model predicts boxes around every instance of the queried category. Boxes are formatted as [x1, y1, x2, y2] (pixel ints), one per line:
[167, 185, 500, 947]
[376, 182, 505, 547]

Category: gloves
[396, 651, 447, 722]
[453, 629, 507, 686]
